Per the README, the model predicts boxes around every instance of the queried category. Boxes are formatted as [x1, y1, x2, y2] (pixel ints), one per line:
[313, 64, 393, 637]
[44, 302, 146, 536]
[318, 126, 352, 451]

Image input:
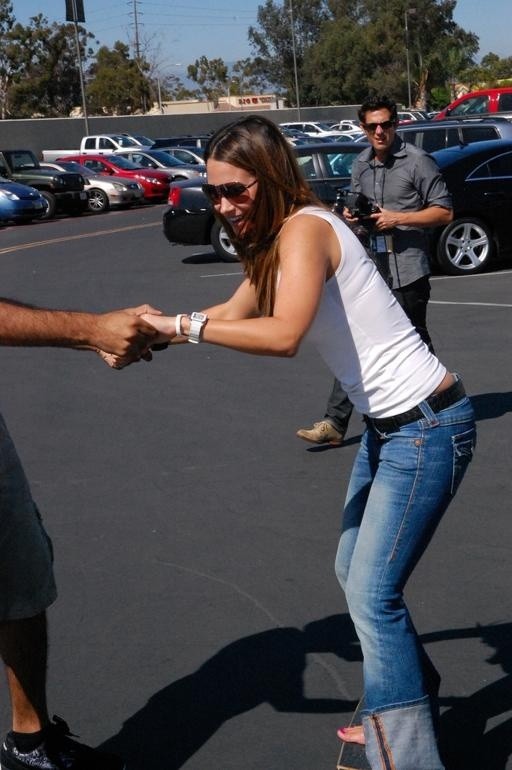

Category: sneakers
[0, 726, 126, 770]
[295, 420, 344, 447]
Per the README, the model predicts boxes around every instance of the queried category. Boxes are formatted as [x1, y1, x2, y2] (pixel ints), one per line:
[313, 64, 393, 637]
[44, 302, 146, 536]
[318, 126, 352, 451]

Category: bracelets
[174, 313, 186, 339]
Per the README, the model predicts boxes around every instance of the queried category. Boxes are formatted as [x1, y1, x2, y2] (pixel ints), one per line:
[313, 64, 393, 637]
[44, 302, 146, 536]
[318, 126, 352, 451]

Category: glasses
[362, 118, 396, 133]
[201, 178, 259, 204]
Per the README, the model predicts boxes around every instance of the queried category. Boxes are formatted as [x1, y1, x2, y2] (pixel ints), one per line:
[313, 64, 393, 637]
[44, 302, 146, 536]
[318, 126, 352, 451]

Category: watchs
[188, 310, 208, 344]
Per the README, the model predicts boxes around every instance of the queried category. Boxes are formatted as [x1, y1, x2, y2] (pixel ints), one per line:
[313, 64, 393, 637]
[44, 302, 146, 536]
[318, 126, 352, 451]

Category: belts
[364, 379, 467, 437]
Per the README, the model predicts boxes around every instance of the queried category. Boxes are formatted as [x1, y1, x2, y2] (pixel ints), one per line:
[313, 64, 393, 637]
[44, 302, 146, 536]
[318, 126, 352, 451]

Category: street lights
[158, 64, 181, 110]
[404, 7, 417, 110]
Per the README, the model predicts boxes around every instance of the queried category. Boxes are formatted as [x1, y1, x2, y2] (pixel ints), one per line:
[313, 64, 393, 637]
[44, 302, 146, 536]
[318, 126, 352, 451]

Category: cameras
[336, 189, 382, 226]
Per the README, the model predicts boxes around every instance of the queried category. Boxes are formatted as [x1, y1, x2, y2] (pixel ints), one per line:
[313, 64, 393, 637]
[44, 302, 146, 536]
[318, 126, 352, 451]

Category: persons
[138, 114, 480, 770]
[0, 296, 164, 770]
[293, 92, 455, 446]
